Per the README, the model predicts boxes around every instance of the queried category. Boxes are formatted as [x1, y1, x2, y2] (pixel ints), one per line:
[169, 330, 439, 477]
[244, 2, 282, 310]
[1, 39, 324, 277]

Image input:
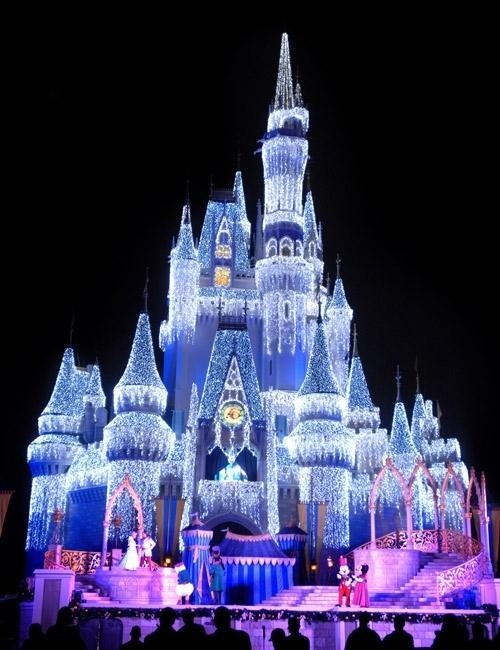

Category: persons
[140, 532, 156, 571]
[21, 606, 499, 650]
[208, 546, 226, 604]
[118, 531, 142, 571]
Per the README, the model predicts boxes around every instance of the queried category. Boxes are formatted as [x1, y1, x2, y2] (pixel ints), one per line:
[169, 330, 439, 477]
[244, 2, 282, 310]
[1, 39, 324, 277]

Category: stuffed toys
[351, 561, 369, 607]
[335, 555, 354, 607]
